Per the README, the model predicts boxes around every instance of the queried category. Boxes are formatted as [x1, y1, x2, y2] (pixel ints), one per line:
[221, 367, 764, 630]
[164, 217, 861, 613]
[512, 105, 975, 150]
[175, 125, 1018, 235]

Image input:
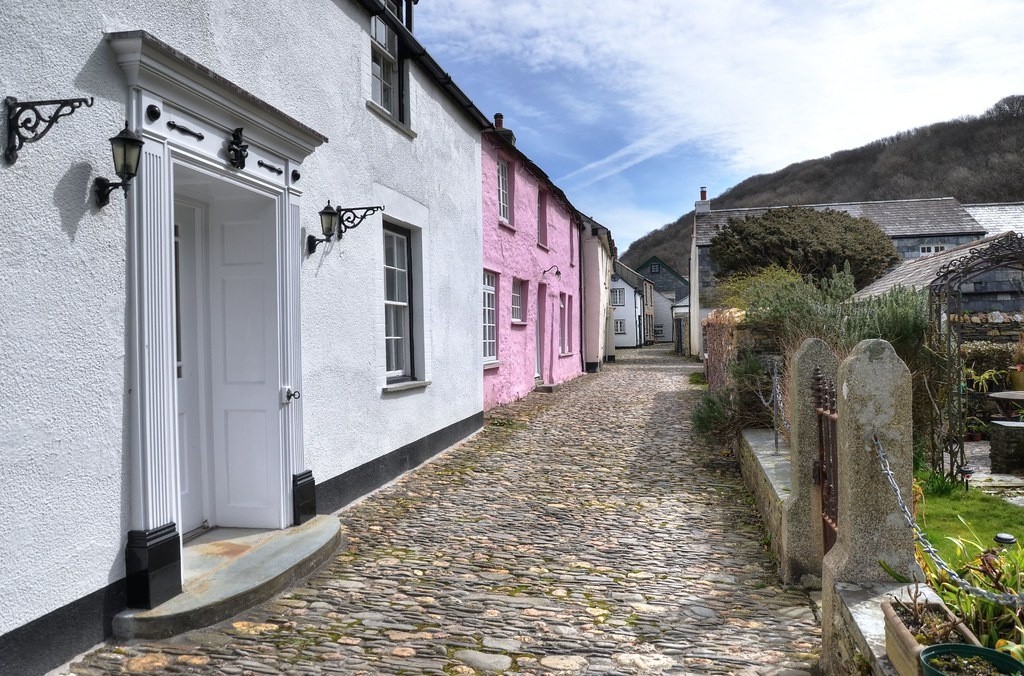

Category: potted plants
[955, 334, 1024, 442]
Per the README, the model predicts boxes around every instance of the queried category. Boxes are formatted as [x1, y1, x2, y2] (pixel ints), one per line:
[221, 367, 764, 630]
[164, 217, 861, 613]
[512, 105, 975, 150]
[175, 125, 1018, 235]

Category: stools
[989, 421, 1024, 474]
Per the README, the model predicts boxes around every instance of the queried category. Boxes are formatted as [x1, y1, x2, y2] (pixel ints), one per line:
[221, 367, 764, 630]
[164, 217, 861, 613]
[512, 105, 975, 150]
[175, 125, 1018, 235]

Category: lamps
[544, 265, 561, 275]
[308, 200, 340, 254]
[95, 121, 145, 207]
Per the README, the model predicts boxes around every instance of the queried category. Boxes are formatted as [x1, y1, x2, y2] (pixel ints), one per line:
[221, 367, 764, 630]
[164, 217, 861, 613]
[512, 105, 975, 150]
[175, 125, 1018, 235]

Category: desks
[989, 391, 1024, 420]
